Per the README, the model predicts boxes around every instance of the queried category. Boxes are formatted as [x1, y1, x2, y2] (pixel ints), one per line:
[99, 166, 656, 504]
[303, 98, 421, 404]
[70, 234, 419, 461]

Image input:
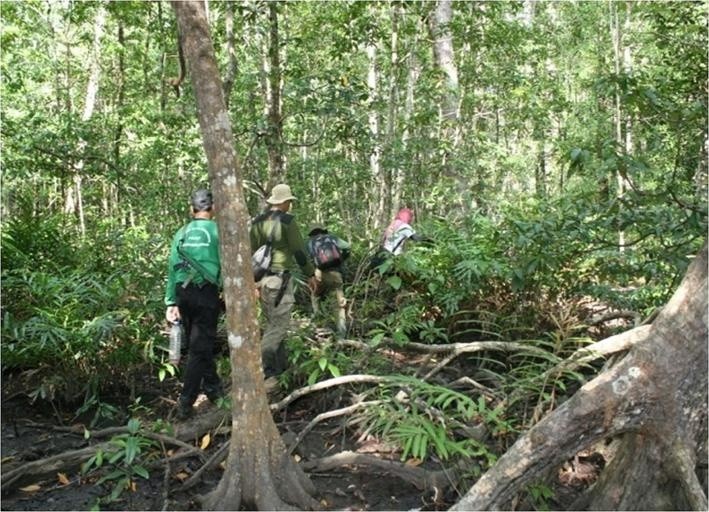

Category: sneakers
[174, 387, 224, 423]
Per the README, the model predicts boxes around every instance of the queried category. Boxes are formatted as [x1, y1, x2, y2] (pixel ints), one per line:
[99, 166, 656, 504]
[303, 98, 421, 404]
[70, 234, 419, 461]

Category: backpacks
[305, 233, 344, 270]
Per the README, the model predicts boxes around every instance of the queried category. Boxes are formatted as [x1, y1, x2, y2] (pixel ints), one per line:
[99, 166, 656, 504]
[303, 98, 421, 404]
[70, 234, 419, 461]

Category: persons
[163, 188, 229, 421]
[246, 185, 318, 379]
[374, 204, 432, 315]
[303, 228, 354, 337]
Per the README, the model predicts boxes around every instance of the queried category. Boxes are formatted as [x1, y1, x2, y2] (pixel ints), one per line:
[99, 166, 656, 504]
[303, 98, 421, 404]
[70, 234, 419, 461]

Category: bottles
[167, 321, 182, 366]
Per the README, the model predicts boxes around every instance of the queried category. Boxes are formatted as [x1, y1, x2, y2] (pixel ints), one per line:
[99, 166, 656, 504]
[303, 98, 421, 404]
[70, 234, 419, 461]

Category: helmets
[190, 188, 214, 212]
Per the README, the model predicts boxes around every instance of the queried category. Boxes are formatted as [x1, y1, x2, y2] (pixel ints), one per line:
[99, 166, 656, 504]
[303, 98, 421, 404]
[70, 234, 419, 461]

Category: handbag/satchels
[370, 246, 396, 269]
[252, 244, 273, 283]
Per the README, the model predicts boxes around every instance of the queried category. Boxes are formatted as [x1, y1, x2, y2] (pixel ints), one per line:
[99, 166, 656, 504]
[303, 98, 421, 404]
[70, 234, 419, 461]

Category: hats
[307, 224, 328, 237]
[266, 183, 298, 205]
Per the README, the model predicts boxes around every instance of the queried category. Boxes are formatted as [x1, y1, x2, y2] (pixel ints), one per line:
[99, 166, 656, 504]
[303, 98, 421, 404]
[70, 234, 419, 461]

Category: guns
[174, 245, 222, 292]
